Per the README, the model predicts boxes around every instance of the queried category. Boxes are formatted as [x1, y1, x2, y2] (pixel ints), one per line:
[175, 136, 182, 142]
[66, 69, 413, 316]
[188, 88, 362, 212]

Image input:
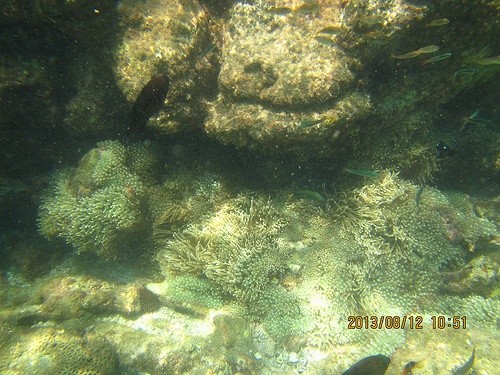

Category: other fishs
[120, 75, 170, 143]
[417, 45, 440, 53]
[310, 35, 335, 45]
[316, 26, 342, 35]
[391, 51, 420, 59]
[401, 357, 426, 375]
[267, 7, 292, 16]
[426, 19, 449, 27]
[294, 4, 321, 13]
[423, 53, 452, 64]
[416, 184, 426, 209]
[450, 344, 476, 375]
[300, 116, 327, 127]
[340, 354, 390, 375]
[365, 29, 384, 38]
[344, 167, 379, 178]
[360, 15, 384, 25]
[450, 69, 475, 85]
[289, 190, 323, 202]
